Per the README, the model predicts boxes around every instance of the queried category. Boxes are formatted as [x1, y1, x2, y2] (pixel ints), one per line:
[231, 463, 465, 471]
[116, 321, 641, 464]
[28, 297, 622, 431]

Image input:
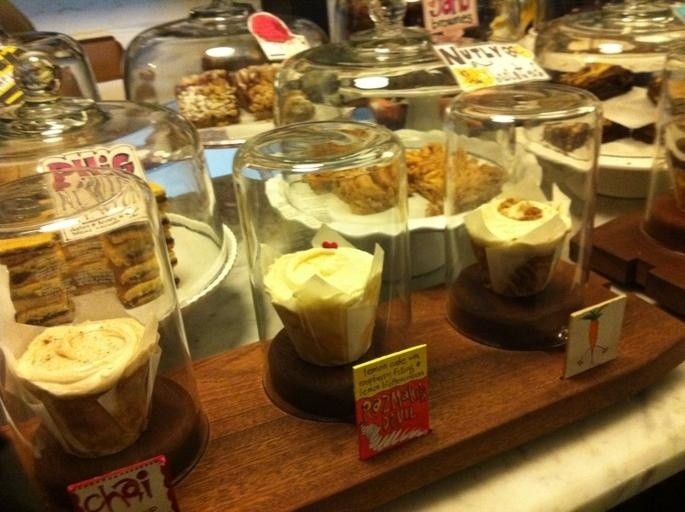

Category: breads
[543, 63, 685, 153]
[0, 187, 180, 327]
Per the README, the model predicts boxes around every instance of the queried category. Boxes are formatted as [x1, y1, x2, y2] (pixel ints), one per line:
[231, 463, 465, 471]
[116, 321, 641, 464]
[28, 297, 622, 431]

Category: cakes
[175, 62, 302, 129]
[268, 246, 383, 367]
[472, 198, 572, 297]
[16, 318, 151, 458]
